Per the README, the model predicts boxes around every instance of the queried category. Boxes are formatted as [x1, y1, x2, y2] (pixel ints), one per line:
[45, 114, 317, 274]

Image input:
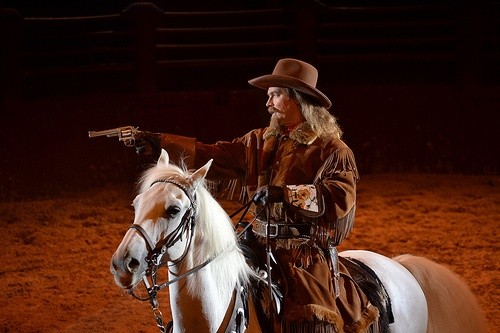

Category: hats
[248, 58, 332, 110]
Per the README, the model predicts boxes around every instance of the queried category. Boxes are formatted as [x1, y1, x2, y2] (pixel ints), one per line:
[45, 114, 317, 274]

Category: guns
[88, 126, 141, 146]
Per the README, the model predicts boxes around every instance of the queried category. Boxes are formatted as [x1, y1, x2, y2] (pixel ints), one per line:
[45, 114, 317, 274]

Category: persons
[133, 59, 382, 331]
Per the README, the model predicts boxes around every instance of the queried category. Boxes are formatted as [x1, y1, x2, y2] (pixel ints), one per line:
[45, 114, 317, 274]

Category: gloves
[126, 130, 160, 156]
[252, 185, 284, 207]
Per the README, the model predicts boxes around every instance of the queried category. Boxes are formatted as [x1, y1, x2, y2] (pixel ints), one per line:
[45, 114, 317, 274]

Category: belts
[252, 219, 309, 239]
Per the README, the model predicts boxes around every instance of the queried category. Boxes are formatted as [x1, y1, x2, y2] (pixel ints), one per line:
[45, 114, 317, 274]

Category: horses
[108, 148, 488, 333]
